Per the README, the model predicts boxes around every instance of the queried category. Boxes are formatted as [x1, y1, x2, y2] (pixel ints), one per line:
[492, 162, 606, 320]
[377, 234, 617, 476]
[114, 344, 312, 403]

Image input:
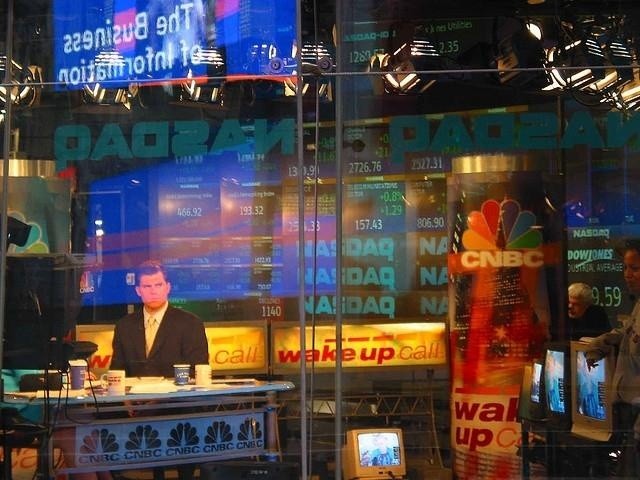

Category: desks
[1, 369, 296, 479]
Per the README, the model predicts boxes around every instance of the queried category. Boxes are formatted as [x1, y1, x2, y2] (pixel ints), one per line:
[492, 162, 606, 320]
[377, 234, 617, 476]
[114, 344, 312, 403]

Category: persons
[585, 237, 640, 480]
[567, 282, 612, 342]
[95, 259, 210, 479]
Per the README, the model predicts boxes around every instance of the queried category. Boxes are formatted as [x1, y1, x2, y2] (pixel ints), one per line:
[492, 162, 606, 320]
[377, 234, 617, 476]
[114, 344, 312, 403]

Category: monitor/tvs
[529, 361, 548, 421]
[570, 340, 640, 444]
[543, 347, 572, 430]
[341, 428, 406, 480]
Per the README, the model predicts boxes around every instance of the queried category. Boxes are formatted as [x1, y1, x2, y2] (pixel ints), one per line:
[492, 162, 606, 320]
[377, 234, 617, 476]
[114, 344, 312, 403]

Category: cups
[69, 360, 87, 390]
[195, 364, 212, 386]
[173, 363, 190, 388]
[100, 370, 126, 398]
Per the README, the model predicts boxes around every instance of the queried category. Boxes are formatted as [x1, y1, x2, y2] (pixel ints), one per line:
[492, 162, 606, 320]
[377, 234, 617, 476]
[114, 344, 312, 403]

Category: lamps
[82, 46, 142, 113]
[0, 54, 47, 113]
[178, 42, 229, 108]
[493, 2, 639, 118]
[270, 42, 337, 102]
[363, 39, 441, 98]
